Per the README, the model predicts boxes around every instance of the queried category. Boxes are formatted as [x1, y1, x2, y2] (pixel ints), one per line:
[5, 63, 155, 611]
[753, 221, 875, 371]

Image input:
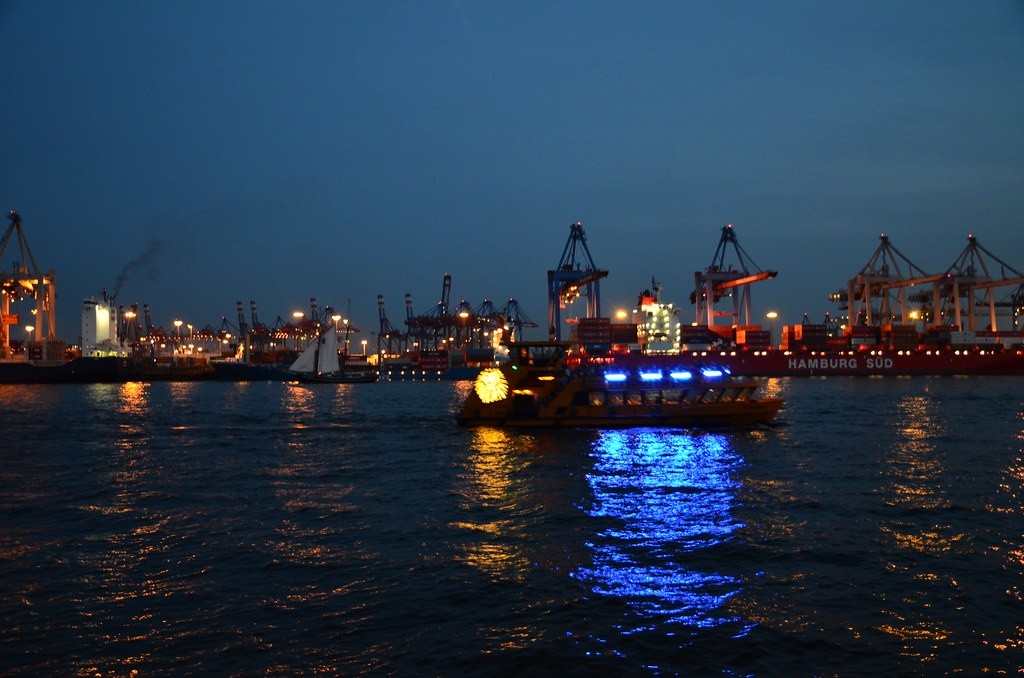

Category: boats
[456, 365, 784, 427]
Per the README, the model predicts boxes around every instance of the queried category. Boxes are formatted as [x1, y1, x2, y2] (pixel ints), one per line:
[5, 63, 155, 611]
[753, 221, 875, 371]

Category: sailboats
[270, 325, 379, 383]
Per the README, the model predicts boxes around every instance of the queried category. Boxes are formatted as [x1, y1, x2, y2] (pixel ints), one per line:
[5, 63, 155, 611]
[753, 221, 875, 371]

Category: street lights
[188, 325, 192, 340]
[176, 322, 180, 339]
[362, 341, 366, 356]
[334, 316, 338, 331]
[27, 326, 32, 339]
[767, 312, 777, 347]
